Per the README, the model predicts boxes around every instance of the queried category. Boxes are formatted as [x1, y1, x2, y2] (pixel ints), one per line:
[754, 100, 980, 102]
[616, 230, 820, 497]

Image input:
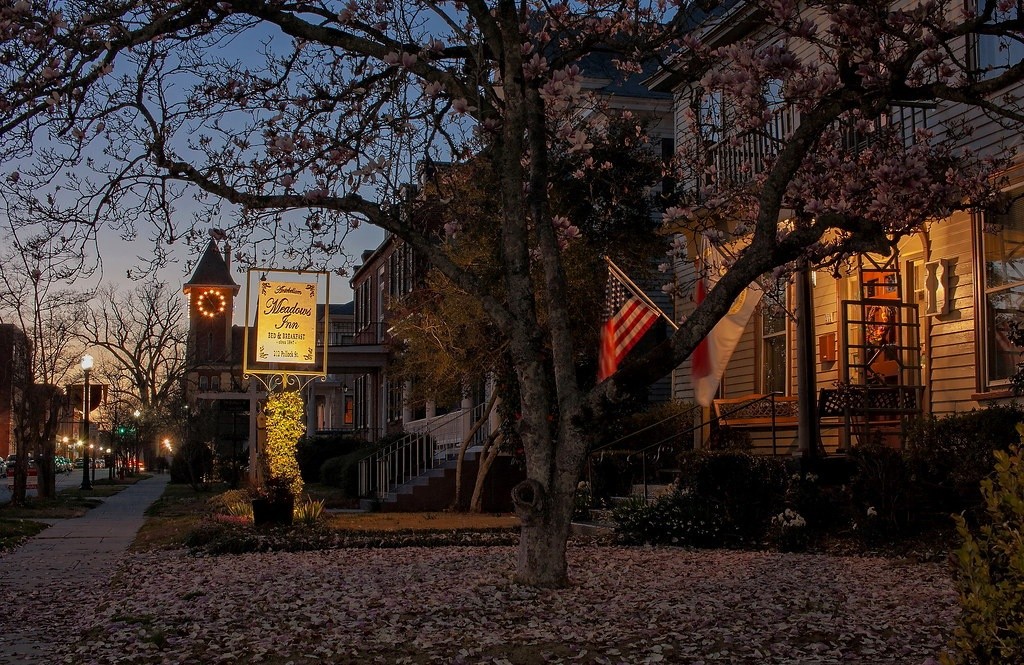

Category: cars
[0, 457, 7, 478]
[28, 456, 74, 475]
[3, 454, 17, 476]
[73, 457, 105, 469]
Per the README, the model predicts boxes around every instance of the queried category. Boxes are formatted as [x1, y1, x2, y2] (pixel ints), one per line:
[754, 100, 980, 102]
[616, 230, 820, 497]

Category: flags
[691, 232, 764, 408]
[596, 263, 661, 384]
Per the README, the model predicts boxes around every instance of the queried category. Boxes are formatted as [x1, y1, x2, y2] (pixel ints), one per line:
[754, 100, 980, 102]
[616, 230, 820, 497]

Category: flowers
[252, 474, 296, 508]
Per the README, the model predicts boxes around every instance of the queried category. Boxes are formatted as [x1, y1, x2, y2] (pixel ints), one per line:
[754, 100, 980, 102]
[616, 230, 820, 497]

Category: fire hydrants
[119, 468, 124, 480]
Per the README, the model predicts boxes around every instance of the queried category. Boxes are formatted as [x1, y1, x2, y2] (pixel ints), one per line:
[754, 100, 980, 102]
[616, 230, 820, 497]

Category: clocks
[198, 289, 227, 318]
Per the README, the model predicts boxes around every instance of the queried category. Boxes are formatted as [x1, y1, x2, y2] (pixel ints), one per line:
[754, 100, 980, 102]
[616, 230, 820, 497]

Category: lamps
[343, 384, 351, 395]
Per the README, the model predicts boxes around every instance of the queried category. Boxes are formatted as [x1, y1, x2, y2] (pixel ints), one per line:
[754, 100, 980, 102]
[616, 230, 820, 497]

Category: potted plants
[251, 499, 295, 526]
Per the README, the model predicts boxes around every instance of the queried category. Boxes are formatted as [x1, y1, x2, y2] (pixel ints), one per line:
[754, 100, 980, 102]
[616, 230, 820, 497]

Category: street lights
[133, 410, 140, 473]
[79, 353, 93, 491]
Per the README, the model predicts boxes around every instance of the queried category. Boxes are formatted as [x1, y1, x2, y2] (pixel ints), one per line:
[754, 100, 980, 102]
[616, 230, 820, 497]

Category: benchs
[712, 392, 829, 452]
[818, 386, 924, 453]
